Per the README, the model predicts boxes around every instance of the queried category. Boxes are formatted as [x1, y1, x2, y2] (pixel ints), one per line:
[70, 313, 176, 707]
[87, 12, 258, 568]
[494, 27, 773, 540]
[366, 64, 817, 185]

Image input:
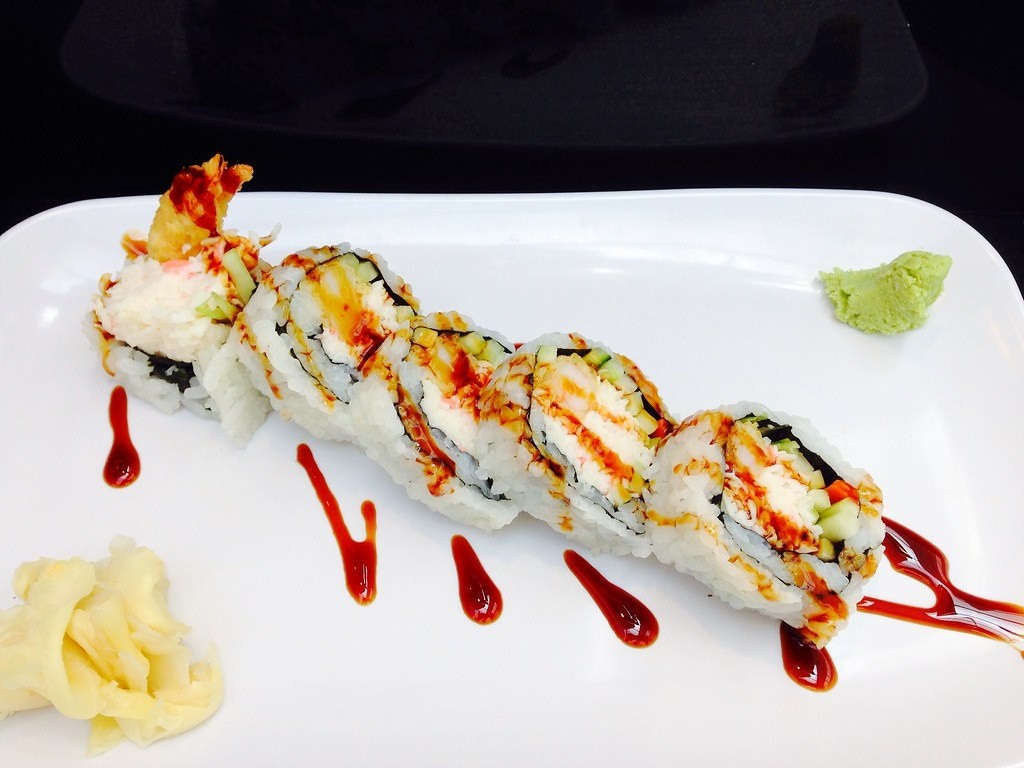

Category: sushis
[87, 155, 885, 649]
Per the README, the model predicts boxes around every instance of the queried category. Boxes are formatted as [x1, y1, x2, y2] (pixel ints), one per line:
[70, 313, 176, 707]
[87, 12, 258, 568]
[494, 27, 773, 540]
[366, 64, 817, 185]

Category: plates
[0, 188, 1024, 768]
